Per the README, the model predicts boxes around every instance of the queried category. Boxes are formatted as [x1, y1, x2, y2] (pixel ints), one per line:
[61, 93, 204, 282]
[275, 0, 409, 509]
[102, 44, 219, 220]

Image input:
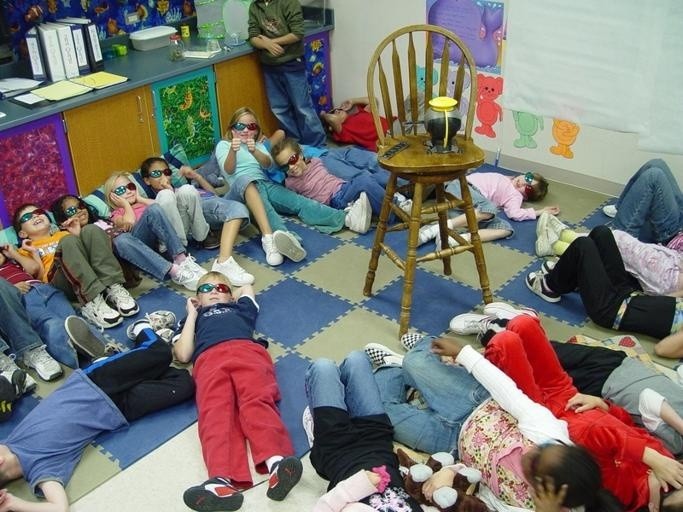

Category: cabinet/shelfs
[299, 30, 334, 120]
[214, 50, 276, 147]
[61, 61, 220, 200]
[0, 110, 81, 234]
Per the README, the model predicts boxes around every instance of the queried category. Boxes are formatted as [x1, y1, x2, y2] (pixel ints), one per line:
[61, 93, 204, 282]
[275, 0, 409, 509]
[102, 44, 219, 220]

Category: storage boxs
[129, 24, 177, 52]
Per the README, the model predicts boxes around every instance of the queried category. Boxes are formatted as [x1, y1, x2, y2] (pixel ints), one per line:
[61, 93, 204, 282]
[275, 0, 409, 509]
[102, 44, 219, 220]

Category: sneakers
[549, 214, 570, 240]
[483, 301, 538, 320]
[126, 310, 177, 342]
[64, 314, 106, 359]
[203, 231, 221, 249]
[364, 342, 405, 367]
[81, 293, 124, 329]
[435, 232, 451, 251]
[0, 375, 15, 422]
[183, 478, 243, 512]
[541, 260, 554, 274]
[400, 332, 427, 351]
[392, 191, 405, 207]
[23, 343, 63, 382]
[302, 404, 315, 450]
[602, 205, 618, 218]
[211, 256, 254, 288]
[449, 313, 494, 343]
[171, 266, 202, 291]
[174, 252, 208, 277]
[0, 370, 25, 400]
[272, 230, 307, 263]
[525, 272, 562, 303]
[399, 198, 412, 215]
[259, 233, 284, 267]
[343, 191, 372, 235]
[266, 456, 303, 501]
[418, 224, 435, 245]
[104, 283, 140, 318]
[155, 328, 175, 346]
[2, 352, 37, 394]
[534, 211, 559, 258]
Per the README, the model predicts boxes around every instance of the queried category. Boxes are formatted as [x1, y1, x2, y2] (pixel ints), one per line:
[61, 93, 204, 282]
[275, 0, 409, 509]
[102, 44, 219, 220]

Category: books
[0, 14, 130, 108]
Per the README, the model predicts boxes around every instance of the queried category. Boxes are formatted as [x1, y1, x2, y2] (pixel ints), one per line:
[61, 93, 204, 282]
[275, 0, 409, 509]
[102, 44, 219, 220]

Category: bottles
[168, 35, 184, 62]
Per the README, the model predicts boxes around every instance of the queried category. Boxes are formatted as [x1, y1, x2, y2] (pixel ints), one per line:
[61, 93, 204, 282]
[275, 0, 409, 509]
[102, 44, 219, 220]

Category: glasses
[197, 282, 232, 297]
[233, 122, 258, 131]
[18, 208, 45, 224]
[329, 108, 343, 114]
[659, 481, 676, 510]
[63, 201, 88, 218]
[114, 183, 136, 196]
[145, 168, 172, 178]
[279, 154, 299, 173]
[524, 172, 534, 196]
[530, 437, 568, 479]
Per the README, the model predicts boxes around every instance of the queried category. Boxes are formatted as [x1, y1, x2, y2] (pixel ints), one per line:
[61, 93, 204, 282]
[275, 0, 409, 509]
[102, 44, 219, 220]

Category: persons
[0, 272, 63, 399]
[171, 270, 302, 511]
[0, 243, 121, 372]
[105, 94, 415, 295]
[1, 309, 197, 512]
[306, 159, 683, 512]
[421, 173, 560, 253]
[13, 204, 140, 330]
[245, 0, 328, 147]
[49, 195, 208, 291]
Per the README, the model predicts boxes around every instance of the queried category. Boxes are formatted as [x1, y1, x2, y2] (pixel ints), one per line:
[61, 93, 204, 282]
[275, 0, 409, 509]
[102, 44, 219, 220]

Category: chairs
[361, 24, 494, 341]
